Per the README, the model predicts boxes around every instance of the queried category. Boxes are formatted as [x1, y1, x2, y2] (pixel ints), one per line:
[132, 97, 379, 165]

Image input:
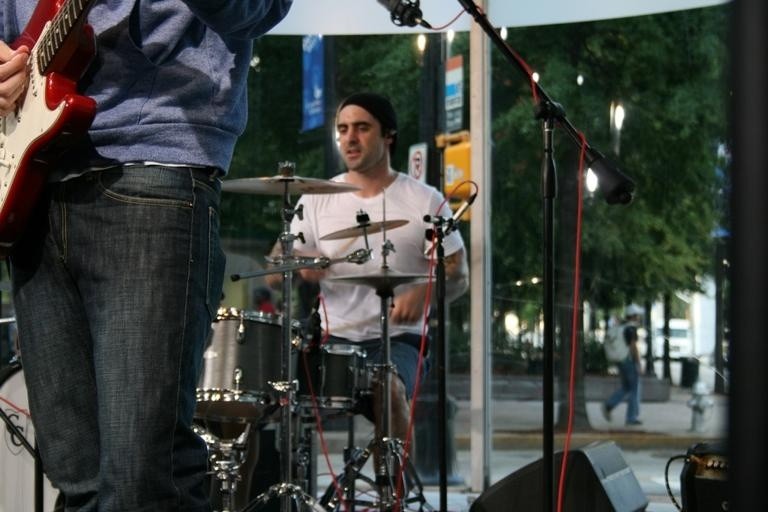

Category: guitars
[0, 0, 98, 261]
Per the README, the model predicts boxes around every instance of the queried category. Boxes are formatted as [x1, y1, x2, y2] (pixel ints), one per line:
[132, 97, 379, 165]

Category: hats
[340, 93, 397, 157]
[625, 305, 645, 317]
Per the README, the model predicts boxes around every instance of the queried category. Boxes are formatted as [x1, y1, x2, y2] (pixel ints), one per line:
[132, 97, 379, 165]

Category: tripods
[326, 289, 437, 511]
[228, 186, 329, 511]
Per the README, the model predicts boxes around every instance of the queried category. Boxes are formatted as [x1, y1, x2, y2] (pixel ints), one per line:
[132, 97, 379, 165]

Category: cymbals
[322, 220, 409, 240]
[326, 267, 448, 290]
[221, 175, 364, 196]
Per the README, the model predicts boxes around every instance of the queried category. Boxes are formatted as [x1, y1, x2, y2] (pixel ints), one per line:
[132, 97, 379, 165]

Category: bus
[654, 317, 690, 360]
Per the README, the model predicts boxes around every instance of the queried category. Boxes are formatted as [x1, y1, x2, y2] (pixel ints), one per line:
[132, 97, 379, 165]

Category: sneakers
[625, 420, 642, 426]
[601, 403, 610, 421]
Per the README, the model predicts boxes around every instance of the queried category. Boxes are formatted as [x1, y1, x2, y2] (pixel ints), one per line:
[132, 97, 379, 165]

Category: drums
[294, 343, 367, 416]
[194, 307, 303, 425]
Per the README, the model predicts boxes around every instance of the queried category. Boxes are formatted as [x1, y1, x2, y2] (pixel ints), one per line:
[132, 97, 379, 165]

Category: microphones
[377, 0, 433, 28]
[424, 192, 477, 257]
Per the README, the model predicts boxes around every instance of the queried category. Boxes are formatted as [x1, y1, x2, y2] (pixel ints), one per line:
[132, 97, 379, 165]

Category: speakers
[679, 442, 730, 511]
[469, 437, 649, 512]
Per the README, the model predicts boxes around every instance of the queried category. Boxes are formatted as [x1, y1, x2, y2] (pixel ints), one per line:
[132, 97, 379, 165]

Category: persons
[0, 0, 294, 510]
[264, 93, 468, 504]
[601, 303, 648, 426]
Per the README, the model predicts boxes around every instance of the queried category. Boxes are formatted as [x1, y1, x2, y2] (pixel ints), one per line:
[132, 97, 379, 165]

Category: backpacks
[605, 323, 637, 362]
